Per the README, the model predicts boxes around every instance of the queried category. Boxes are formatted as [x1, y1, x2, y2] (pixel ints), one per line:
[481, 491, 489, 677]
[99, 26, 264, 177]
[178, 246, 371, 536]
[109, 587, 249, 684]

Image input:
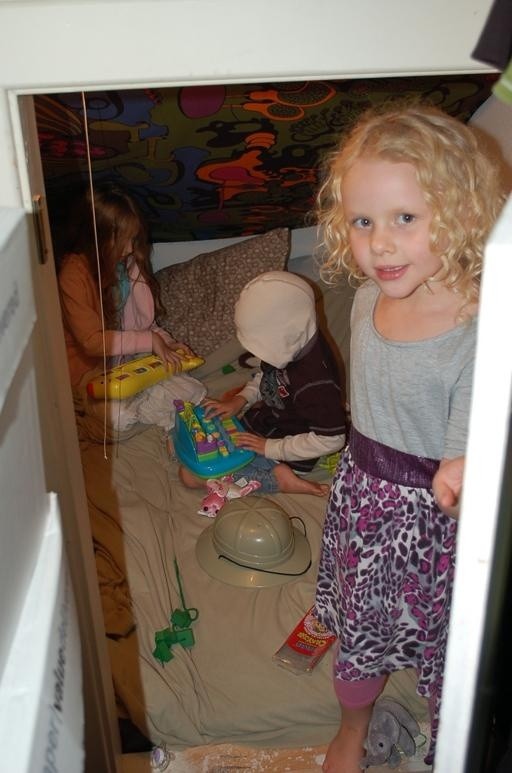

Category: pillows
[154, 226, 295, 361]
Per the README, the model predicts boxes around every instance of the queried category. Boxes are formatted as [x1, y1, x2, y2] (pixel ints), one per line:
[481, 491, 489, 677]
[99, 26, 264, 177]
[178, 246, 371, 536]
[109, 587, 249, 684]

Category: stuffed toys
[360, 693, 428, 771]
[195, 472, 263, 517]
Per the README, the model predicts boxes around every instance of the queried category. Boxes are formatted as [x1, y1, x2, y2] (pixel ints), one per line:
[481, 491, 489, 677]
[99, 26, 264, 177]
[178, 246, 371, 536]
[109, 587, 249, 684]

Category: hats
[196, 494, 313, 589]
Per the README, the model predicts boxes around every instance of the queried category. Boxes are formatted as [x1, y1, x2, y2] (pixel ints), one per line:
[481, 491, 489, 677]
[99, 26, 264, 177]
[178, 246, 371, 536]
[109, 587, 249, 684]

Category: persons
[52, 179, 207, 448]
[178, 270, 348, 499]
[307, 106, 512, 771]
